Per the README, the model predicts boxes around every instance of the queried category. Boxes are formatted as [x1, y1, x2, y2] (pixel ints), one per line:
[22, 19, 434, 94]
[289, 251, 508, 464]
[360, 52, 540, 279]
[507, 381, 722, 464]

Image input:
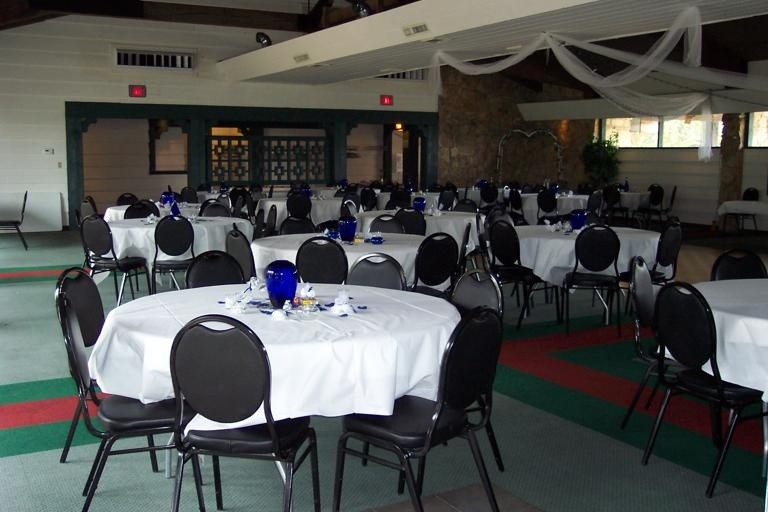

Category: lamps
[255, 31, 272, 49]
[353, 0, 370, 18]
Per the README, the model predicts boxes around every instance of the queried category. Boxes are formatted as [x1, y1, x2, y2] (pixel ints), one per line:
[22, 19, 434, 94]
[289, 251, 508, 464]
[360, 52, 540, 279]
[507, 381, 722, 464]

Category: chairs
[641, 280, 768, 499]
[709, 245, 768, 281]
[741, 187, 759, 232]
[0, 191, 29, 250]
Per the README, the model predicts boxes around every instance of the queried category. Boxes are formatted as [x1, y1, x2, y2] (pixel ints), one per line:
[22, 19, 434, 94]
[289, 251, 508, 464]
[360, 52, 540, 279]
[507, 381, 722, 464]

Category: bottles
[624, 176, 629, 192]
[296, 299, 320, 321]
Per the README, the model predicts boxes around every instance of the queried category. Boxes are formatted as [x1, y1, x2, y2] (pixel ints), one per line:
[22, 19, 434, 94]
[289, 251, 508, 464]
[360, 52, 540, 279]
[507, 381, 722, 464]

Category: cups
[552, 184, 560, 194]
[266, 260, 299, 310]
[412, 197, 426, 215]
[337, 215, 357, 243]
[371, 237, 384, 244]
[570, 209, 588, 230]
[479, 180, 488, 189]
[161, 192, 174, 206]
[341, 179, 348, 189]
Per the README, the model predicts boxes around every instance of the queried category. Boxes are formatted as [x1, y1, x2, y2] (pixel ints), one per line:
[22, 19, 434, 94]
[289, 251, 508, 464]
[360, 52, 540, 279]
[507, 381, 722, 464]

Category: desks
[716, 200, 768, 232]
